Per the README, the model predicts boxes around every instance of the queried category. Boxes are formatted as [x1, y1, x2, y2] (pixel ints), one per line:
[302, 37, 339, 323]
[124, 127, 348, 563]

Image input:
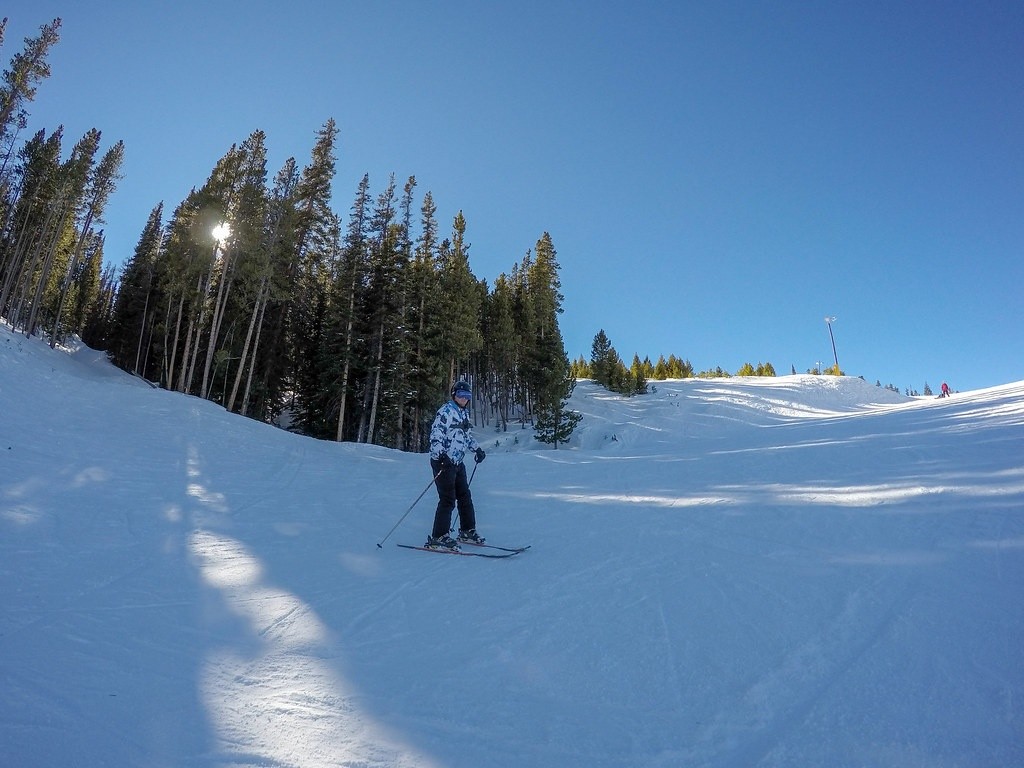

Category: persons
[935, 392, 943, 399]
[424, 380, 485, 554]
[942, 382, 950, 398]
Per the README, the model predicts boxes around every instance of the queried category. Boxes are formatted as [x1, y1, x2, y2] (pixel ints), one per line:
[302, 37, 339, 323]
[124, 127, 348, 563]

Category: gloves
[440, 458, 450, 470]
[474, 448, 485, 463]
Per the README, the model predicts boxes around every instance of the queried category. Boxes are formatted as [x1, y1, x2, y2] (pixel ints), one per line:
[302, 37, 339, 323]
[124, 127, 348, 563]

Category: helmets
[451, 381, 474, 408]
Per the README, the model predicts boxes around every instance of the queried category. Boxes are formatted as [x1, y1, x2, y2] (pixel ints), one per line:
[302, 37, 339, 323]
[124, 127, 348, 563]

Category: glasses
[456, 390, 472, 400]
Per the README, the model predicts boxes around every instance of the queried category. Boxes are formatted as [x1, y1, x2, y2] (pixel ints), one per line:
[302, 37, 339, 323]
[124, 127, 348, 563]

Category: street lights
[816, 360, 822, 374]
[825, 317, 838, 364]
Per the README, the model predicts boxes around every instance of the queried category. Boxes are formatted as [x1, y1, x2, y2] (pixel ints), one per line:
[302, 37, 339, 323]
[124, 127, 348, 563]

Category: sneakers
[456, 528, 486, 545]
[424, 534, 461, 553]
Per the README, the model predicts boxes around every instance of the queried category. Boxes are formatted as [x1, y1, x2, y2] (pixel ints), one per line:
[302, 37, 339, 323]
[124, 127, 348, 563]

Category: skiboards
[396, 536, 532, 559]
